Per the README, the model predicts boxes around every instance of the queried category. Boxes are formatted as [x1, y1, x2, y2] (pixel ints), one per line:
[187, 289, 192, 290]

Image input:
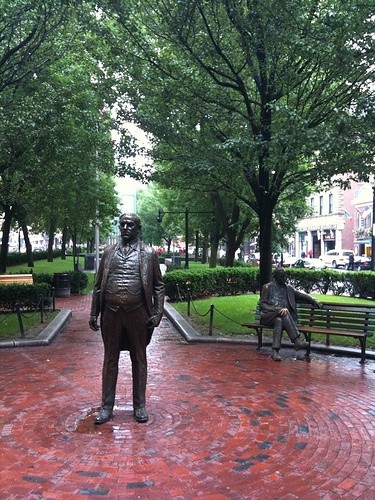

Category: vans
[319, 249, 355, 269]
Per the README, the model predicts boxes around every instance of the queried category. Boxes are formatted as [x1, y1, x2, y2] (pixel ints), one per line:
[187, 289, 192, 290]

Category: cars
[345, 255, 372, 272]
[221, 252, 325, 271]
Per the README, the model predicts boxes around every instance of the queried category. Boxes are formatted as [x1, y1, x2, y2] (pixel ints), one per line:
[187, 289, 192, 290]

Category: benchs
[241, 298, 375, 364]
[0, 274, 33, 286]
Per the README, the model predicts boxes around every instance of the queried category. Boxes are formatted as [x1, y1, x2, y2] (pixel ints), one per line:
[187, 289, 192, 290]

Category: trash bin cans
[171, 256, 181, 268]
[84, 254, 95, 270]
[53, 272, 70, 298]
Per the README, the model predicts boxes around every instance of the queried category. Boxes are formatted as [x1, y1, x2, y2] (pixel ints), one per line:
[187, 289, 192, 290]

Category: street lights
[156, 207, 217, 270]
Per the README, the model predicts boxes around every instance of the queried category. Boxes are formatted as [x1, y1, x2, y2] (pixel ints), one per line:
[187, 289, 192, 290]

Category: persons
[308, 249, 313, 258]
[89, 212, 165, 425]
[260, 267, 320, 361]
[347, 252, 354, 271]
